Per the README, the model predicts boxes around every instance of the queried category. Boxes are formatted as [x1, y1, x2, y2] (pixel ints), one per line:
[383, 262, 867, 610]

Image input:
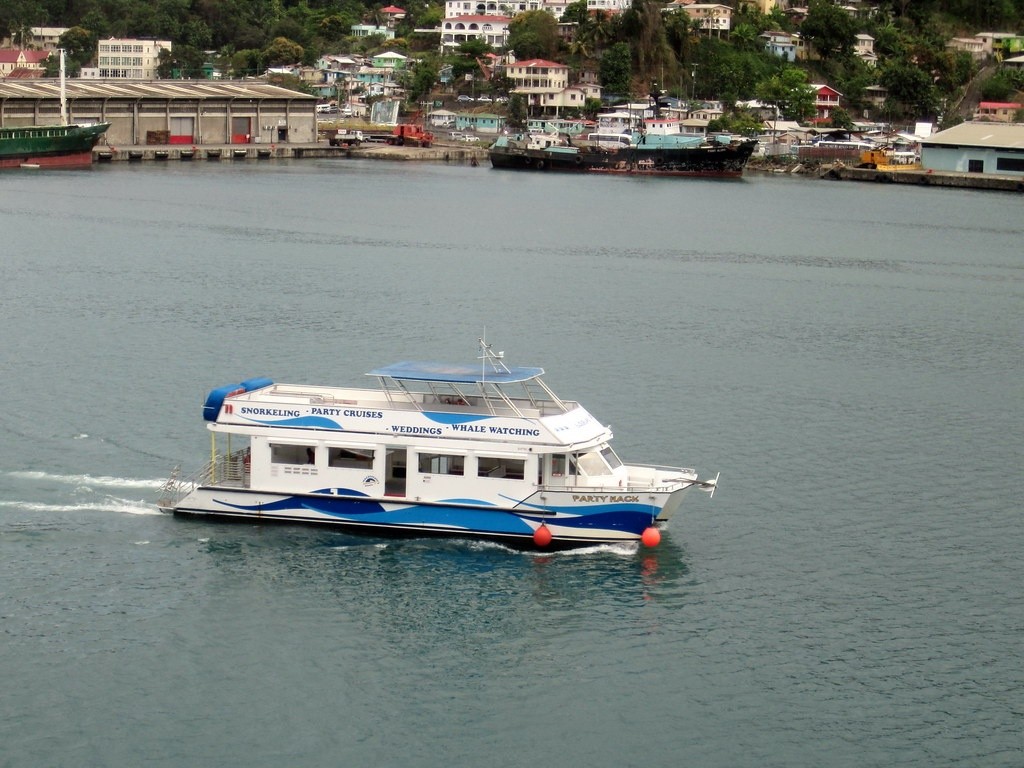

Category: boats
[488, 131, 759, 178]
[0, 120, 113, 169]
[158, 324, 722, 556]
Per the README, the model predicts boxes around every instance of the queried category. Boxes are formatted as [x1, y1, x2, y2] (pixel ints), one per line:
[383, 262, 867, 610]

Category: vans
[894, 151, 916, 165]
[448, 132, 464, 141]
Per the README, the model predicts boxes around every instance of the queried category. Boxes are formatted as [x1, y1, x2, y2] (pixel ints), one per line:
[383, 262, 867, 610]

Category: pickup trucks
[460, 134, 480, 142]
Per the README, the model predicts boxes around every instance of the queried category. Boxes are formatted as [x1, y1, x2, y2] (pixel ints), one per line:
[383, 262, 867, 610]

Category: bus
[588, 132, 633, 150]
[817, 141, 871, 152]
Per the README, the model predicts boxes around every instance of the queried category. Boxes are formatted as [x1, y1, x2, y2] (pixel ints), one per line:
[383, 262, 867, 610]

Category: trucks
[328, 130, 363, 147]
